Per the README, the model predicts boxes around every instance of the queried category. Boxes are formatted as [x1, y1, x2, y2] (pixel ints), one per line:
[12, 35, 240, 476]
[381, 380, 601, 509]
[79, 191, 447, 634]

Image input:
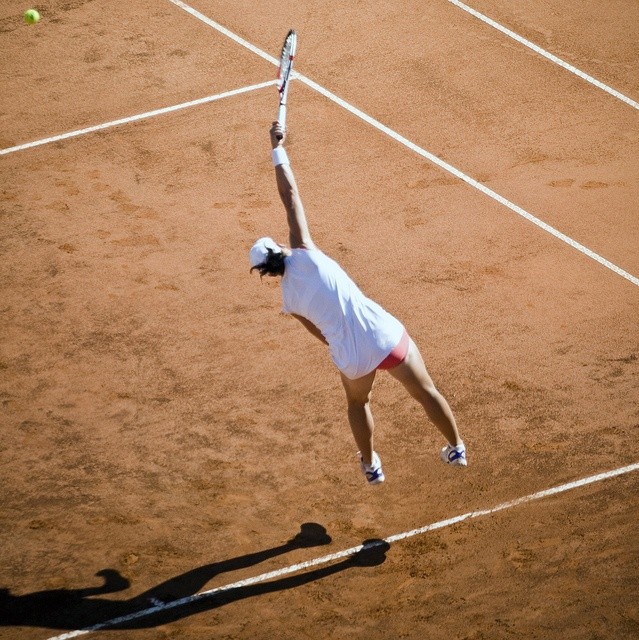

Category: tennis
[23, 9, 39, 24]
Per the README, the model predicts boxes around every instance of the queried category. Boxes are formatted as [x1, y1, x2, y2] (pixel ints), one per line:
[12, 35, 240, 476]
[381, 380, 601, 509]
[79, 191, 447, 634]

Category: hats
[248, 237, 282, 267]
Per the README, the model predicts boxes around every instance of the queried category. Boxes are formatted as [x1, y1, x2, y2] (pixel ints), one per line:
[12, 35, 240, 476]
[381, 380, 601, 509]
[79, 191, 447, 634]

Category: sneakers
[440, 445, 467, 467]
[360, 451, 385, 485]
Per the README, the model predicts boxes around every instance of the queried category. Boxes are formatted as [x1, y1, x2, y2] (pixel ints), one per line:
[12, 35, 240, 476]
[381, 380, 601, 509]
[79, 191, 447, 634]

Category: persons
[247, 119, 468, 485]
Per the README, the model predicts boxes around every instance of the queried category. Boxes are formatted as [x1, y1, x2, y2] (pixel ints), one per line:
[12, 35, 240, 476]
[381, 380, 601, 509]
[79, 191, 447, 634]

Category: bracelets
[271, 146, 289, 167]
